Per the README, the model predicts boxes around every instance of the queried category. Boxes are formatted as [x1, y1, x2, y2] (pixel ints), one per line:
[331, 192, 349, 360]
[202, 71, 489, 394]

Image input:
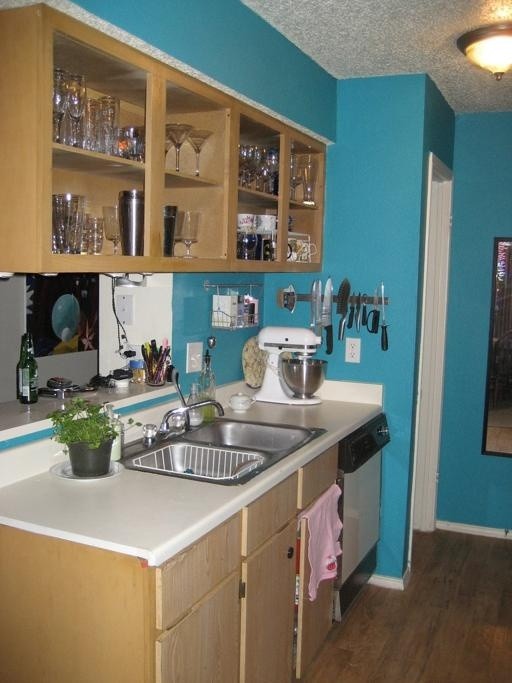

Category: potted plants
[46, 396, 122, 478]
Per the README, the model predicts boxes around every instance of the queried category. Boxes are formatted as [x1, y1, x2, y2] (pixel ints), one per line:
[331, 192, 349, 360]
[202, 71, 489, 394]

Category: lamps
[456, 22, 512, 82]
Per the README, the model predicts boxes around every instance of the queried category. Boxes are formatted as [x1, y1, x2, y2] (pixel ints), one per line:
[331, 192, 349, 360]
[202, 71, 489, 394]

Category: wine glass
[179, 211, 202, 260]
[289, 154, 318, 206]
[164, 123, 214, 177]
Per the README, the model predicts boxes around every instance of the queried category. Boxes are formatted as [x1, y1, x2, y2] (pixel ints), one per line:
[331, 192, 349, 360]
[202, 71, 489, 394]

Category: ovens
[335, 413, 392, 622]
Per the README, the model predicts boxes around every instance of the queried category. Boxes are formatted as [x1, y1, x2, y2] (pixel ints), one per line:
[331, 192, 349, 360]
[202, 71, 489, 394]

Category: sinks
[178, 417, 310, 455]
[124, 440, 267, 488]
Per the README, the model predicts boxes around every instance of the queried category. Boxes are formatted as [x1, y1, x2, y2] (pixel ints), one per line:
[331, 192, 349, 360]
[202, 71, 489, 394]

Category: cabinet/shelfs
[233, 101, 327, 273]
[0, 511, 245, 683]
[1, 5, 235, 273]
[243, 444, 343, 683]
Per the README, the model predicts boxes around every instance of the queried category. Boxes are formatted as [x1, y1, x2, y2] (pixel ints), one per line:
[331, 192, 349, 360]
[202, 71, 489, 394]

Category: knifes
[309, 277, 361, 354]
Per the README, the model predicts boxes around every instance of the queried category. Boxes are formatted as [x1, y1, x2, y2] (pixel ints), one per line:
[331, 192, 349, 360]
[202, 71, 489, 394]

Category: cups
[163, 205, 178, 258]
[145, 359, 166, 386]
[53, 68, 119, 155]
[52, 190, 144, 256]
[236, 214, 317, 262]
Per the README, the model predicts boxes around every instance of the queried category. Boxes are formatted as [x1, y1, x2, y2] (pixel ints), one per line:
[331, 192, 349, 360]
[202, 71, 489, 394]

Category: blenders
[252, 325, 328, 405]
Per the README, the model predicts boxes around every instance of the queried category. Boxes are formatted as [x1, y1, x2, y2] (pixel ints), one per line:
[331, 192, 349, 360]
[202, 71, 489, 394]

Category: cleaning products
[186, 383, 203, 427]
[199, 347, 215, 421]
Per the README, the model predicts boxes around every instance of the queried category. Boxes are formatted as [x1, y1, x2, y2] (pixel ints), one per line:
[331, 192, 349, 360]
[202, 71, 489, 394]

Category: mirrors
[481, 236, 511, 458]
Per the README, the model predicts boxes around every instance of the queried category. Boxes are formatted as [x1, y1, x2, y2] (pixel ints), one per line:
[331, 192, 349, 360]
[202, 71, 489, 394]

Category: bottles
[117, 125, 142, 160]
[239, 143, 278, 193]
[142, 424, 157, 449]
[129, 359, 143, 383]
[199, 355, 216, 423]
[17, 333, 39, 404]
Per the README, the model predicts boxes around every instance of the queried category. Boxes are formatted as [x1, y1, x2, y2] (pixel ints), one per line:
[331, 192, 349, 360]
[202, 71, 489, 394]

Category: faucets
[156, 399, 224, 441]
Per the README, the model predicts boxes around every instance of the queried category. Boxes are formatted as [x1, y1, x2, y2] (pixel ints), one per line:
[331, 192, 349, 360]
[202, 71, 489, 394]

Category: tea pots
[224, 393, 256, 413]
